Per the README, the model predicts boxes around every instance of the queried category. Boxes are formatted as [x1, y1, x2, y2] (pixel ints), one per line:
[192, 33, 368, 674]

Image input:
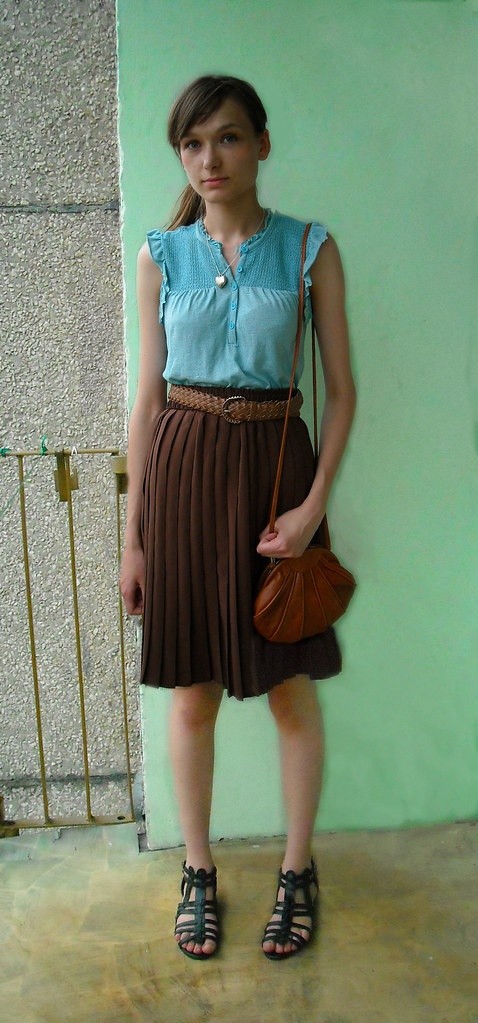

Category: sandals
[175, 858, 221, 960]
[261, 856, 320, 961]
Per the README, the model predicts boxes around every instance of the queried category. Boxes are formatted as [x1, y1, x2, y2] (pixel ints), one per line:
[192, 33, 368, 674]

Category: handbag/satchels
[253, 549, 358, 644]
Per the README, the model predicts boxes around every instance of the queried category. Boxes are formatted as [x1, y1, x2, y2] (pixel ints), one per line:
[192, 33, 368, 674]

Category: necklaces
[206, 214, 265, 288]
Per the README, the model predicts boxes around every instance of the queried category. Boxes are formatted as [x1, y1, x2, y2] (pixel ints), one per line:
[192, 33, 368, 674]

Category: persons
[116, 75, 358, 961]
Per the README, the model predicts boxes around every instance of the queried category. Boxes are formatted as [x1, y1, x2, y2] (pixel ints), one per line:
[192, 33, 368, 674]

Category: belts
[168, 384, 305, 424]
